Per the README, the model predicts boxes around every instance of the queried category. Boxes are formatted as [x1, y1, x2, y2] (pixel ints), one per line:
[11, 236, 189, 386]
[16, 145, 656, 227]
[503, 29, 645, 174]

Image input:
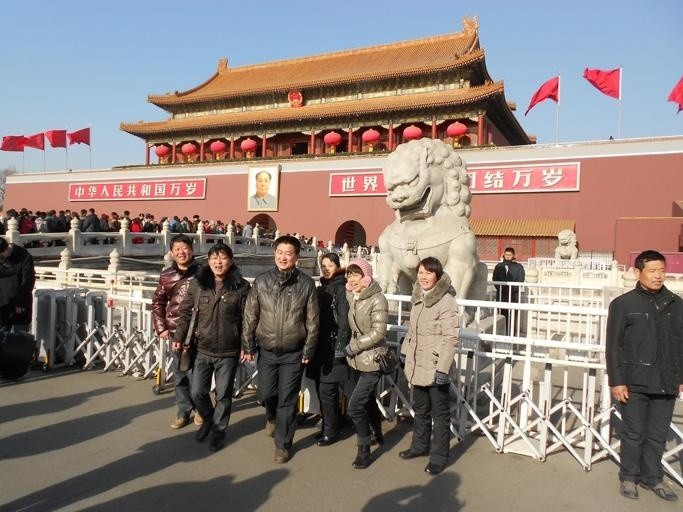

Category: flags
[524, 74, 559, 114]
[667, 77, 683, 113]
[583, 67, 622, 98]
[67, 128, 90, 146]
[44, 130, 66, 148]
[23, 133, 44, 150]
[1, 134, 25, 151]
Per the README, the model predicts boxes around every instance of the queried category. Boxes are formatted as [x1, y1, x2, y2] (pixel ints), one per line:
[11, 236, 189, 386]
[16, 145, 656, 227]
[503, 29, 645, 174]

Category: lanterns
[182, 143, 197, 162]
[156, 145, 170, 163]
[240, 138, 257, 157]
[447, 122, 467, 147]
[362, 128, 381, 153]
[403, 125, 422, 141]
[324, 130, 342, 154]
[210, 139, 226, 158]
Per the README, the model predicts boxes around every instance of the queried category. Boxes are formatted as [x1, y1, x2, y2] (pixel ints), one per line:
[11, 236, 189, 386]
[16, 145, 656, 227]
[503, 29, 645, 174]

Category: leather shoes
[621, 481, 639, 498]
[425, 463, 444, 475]
[639, 480, 677, 501]
[317, 437, 336, 446]
[399, 450, 422, 459]
[316, 431, 324, 439]
[209, 430, 224, 452]
[195, 422, 210, 442]
[265, 420, 275, 435]
[275, 447, 289, 463]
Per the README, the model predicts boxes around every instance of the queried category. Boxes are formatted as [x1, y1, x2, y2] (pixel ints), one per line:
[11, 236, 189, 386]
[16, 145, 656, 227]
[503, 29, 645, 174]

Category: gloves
[434, 370, 448, 384]
[342, 346, 352, 358]
[399, 354, 406, 364]
[334, 345, 344, 360]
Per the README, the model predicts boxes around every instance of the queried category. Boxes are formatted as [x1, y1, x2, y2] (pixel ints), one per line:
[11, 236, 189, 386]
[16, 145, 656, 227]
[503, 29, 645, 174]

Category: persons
[305, 252, 350, 446]
[605, 250, 683, 502]
[341, 259, 388, 468]
[249, 170, 276, 208]
[398, 256, 461, 476]
[0, 237, 35, 334]
[492, 248, 525, 326]
[152, 235, 204, 430]
[241, 235, 321, 463]
[168, 242, 252, 453]
[0, 207, 381, 254]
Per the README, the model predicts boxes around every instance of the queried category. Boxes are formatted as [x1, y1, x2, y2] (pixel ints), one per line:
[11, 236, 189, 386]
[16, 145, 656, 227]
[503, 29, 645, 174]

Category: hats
[345, 260, 372, 291]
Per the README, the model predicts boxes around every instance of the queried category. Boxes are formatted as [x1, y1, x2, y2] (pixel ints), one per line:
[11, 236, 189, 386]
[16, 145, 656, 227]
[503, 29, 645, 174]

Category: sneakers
[169, 418, 189, 429]
[193, 409, 203, 426]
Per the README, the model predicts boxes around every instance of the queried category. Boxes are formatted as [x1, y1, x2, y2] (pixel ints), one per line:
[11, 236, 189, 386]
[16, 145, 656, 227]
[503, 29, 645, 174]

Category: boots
[369, 422, 383, 447]
[353, 435, 371, 468]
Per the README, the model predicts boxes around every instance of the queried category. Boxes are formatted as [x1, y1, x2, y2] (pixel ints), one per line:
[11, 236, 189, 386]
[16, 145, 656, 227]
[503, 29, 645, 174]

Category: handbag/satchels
[351, 307, 399, 375]
[178, 280, 202, 373]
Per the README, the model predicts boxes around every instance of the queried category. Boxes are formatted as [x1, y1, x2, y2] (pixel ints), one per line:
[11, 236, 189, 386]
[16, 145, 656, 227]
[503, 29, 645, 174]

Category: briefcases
[0, 314, 36, 380]
